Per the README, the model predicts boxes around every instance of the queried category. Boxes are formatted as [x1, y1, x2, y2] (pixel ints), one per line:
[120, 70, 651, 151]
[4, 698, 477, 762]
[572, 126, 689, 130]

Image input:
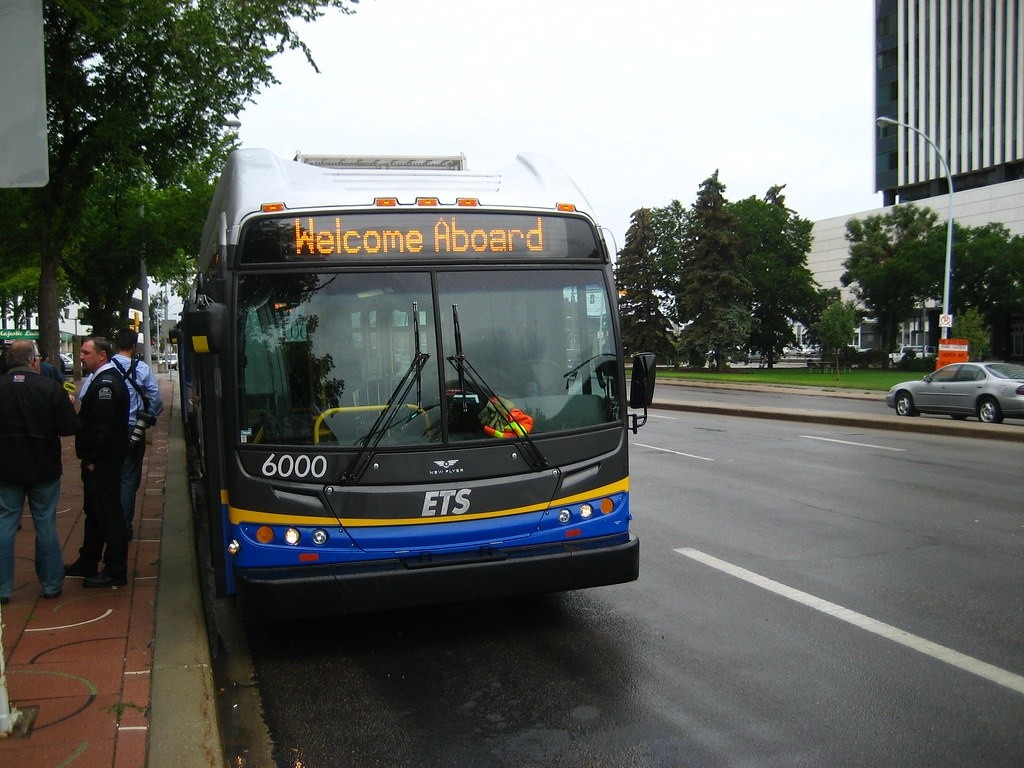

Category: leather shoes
[64, 559, 98, 578]
[82, 572, 127, 587]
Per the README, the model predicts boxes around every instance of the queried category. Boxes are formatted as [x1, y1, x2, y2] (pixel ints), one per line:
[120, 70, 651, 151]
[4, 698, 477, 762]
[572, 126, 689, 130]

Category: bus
[170, 136, 657, 624]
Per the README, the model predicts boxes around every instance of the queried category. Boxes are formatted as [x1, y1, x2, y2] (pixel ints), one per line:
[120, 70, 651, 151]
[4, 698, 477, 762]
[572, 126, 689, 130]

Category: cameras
[131, 411, 157, 441]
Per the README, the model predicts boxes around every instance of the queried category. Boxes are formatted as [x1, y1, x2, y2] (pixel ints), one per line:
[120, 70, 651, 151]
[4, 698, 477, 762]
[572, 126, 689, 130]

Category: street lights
[875, 117, 954, 339]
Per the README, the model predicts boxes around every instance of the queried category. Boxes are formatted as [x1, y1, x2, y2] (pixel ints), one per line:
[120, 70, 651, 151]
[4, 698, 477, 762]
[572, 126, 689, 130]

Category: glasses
[35, 355, 42, 359]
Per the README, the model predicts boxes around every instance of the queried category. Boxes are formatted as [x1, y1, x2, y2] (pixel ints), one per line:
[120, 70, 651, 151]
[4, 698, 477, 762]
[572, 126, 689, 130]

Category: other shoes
[43, 586, 61, 599]
[0, 597, 8, 604]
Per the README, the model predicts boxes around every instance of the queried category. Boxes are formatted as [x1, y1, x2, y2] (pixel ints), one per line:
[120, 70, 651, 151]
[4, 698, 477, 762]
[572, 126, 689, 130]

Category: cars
[885, 361, 1024, 424]
[60, 354, 83, 371]
[162, 354, 178, 370]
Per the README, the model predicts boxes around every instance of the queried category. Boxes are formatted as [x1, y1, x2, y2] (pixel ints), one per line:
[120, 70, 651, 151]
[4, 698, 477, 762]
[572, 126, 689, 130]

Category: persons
[0, 329, 165, 604]
